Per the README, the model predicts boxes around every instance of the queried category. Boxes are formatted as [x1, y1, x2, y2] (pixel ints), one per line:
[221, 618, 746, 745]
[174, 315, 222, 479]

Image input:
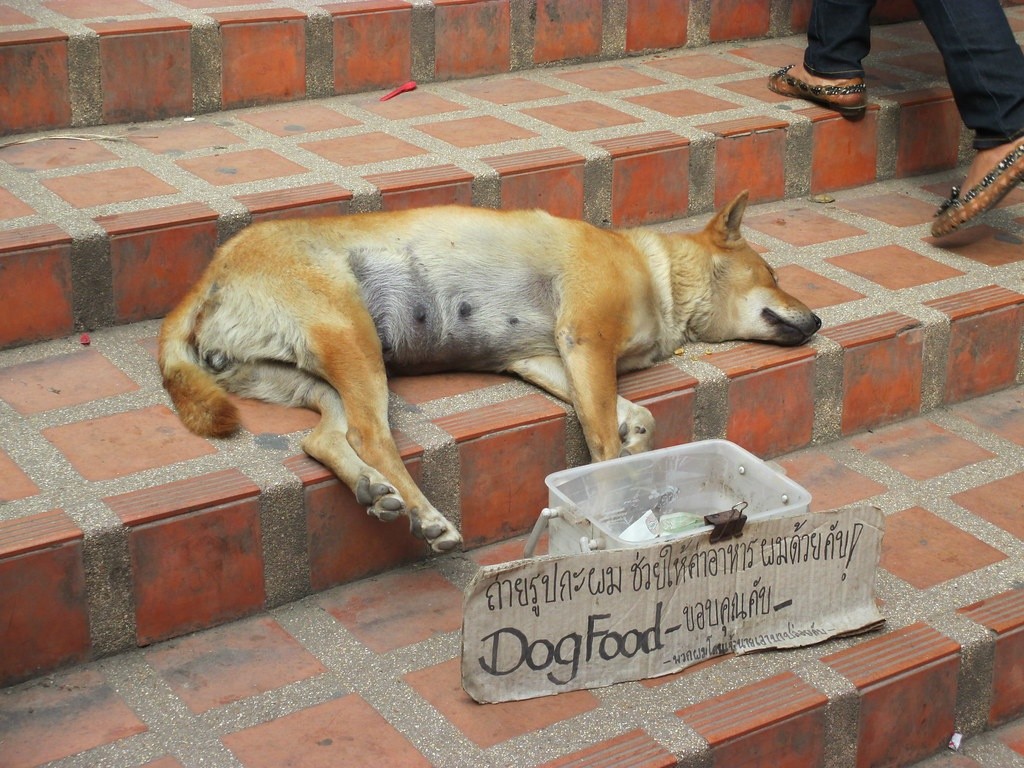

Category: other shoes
[931, 143, 1024, 238]
[767, 64, 866, 118]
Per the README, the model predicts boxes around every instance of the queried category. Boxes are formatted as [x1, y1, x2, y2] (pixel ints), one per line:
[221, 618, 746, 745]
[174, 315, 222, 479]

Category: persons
[766, 0, 1024, 238]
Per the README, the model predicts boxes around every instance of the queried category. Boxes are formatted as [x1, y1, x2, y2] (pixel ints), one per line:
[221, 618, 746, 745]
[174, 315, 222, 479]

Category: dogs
[157, 188, 824, 554]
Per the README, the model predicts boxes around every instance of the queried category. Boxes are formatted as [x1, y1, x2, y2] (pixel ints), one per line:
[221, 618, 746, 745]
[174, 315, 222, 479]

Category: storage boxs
[524, 439, 812, 556]
